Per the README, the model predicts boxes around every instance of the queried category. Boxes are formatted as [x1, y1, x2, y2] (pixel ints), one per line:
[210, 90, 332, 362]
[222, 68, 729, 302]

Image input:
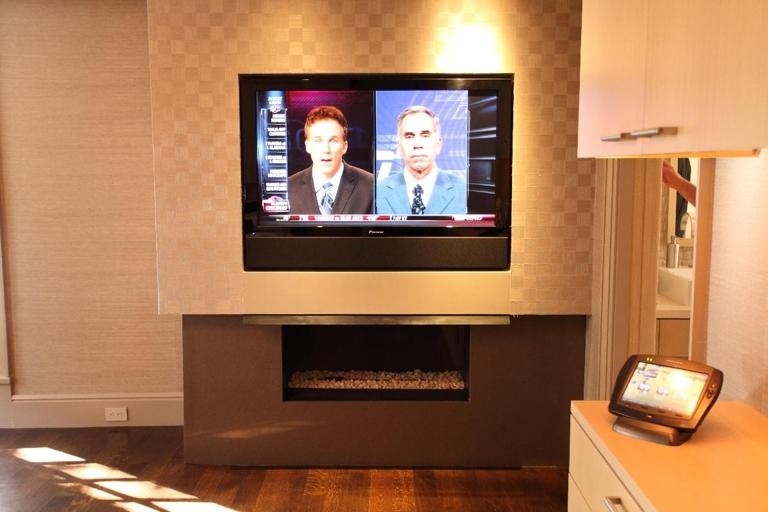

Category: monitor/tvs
[607, 353, 723, 432]
[238, 72, 515, 272]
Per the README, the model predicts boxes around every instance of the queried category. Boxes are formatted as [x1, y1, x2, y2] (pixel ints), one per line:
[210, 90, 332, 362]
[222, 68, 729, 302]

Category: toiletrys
[667, 235, 679, 269]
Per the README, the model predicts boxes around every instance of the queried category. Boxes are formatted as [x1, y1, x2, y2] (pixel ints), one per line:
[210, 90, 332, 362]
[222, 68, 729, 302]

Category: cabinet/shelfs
[565, 400, 766, 512]
[575, 1, 767, 160]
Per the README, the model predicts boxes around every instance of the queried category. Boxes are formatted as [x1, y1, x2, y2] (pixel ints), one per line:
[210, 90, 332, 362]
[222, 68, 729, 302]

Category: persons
[662, 161, 696, 207]
[376, 104, 469, 213]
[289, 105, 373, 213]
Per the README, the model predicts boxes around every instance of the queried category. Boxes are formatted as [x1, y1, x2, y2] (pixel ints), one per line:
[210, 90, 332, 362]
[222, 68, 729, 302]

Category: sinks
[658, 266, 694, 302]
